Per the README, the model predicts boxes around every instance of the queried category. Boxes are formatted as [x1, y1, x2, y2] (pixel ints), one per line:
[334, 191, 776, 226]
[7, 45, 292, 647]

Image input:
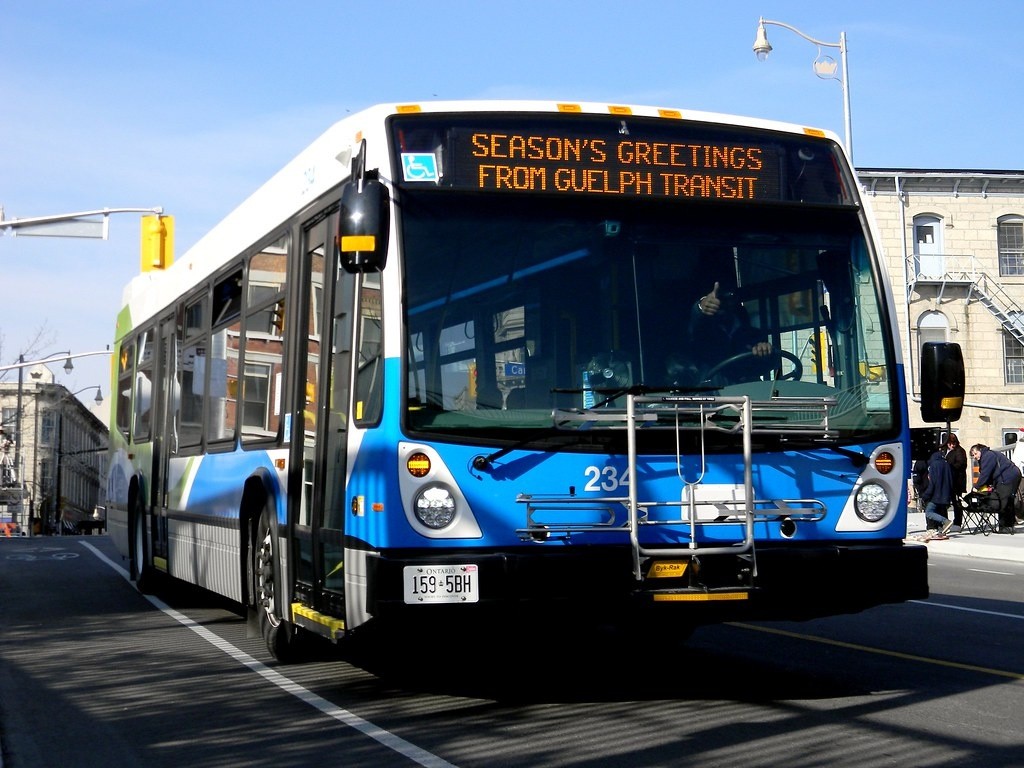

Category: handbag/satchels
[963, 490, 1000, 512]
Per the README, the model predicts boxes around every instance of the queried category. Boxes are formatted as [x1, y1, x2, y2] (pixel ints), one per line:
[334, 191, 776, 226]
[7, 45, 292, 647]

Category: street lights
[752, 15, 868, 418]
[11, 350, 74, 536]
[30, 385, 103, 539]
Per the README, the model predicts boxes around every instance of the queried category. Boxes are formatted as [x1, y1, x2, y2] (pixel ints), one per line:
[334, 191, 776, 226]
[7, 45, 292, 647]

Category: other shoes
[998, 527, 1013, 533]
[950, 525, 961, 532]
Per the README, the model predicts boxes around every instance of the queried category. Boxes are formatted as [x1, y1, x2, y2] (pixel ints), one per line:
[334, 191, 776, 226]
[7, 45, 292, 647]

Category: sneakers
[931, 532, 946, 540]
[942, 519, 953, 534]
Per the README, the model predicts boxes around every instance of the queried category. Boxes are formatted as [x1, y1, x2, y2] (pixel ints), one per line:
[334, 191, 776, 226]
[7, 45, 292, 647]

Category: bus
[107, 100, 966, 665]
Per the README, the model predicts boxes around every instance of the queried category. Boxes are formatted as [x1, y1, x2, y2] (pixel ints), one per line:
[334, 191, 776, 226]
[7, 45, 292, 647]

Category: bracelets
[698, 295, 708, 310]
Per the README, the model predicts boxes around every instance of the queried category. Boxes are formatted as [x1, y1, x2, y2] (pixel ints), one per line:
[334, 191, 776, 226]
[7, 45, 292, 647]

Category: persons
[912, 459, 949, 531]
[969, 443, 1021, 534]
[920, 452, 954, 538]
[940, 433, 968, 533]
[670, 272, 773, 392]
[476, 386, 505, 411]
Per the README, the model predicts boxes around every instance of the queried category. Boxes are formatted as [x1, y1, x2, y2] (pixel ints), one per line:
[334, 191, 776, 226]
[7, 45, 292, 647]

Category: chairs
[505, 356, 571, 411]
